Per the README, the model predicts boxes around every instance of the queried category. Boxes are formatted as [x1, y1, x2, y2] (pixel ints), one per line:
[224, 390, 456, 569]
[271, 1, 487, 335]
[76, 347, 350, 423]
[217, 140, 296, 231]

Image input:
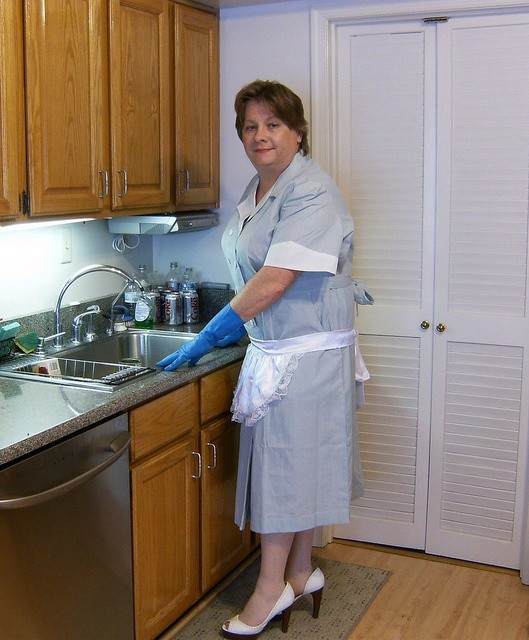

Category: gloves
[214, 323, 247, 348]
[156, 302, 246, 371]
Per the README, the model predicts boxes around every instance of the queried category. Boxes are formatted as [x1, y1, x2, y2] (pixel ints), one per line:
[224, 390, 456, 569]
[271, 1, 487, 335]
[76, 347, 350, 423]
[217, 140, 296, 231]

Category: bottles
[166, 262, 180, 292]
[153, 270, 166, 293]
[179, 268, 199, 291]
[125, 280, 141, 319]
[135, 265, 153, 292]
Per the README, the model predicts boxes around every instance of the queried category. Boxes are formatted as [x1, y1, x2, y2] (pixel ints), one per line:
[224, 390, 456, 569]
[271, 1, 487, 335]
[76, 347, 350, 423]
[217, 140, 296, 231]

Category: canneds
[183, 293, 200, 324]
[156, 286, 163, 295]
[146, 291, 161, 323]
[165, 293, 183, 327]
[161, 290, 174, 321]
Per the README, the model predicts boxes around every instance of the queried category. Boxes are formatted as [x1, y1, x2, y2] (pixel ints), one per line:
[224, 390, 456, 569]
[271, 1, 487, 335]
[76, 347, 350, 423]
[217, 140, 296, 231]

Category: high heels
[221, 581, 295, 640]
[275, 567, 325, 619]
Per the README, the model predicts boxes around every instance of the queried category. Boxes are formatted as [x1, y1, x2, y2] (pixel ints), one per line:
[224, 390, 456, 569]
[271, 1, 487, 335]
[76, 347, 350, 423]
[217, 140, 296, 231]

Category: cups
[119, 359, 142, 371]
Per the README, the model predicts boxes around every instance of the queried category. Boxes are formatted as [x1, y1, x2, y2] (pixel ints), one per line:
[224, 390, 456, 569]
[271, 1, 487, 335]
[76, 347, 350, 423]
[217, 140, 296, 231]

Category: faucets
[110, 278, 144, 333]
[51, 263, 134, 348]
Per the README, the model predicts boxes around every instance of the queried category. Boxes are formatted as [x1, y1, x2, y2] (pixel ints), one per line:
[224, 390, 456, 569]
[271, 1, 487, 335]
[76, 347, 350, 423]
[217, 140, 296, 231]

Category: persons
[156, 79, 373, 639]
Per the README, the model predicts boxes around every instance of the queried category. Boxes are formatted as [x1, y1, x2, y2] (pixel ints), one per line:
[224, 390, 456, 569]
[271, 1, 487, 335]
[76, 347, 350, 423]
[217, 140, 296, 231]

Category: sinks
[0, 326, 218, 393]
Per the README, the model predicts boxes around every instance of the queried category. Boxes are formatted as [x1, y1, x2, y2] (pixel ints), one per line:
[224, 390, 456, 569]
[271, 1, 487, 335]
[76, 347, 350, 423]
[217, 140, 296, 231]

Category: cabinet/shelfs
[0, 0, 27, 224]
[20, 0, 171, 216]
[174, 0, 220, 213]
[128, 361, 255, 640]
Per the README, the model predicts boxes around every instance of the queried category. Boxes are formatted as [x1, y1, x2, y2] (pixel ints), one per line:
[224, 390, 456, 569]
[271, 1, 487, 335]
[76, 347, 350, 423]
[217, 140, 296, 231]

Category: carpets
[171, 553, 392, 640]
